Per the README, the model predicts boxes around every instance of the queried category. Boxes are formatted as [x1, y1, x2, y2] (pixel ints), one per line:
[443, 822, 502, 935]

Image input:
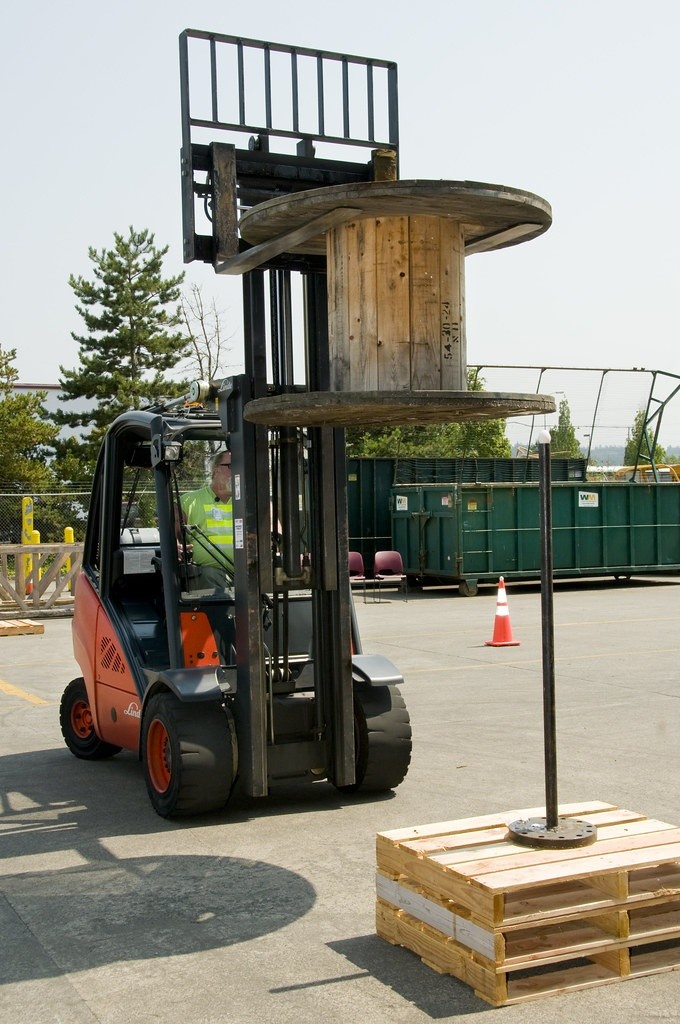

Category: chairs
[372, 551, 408, 604]
[149, 547, 197, 592]
[349, 552, 367, 605]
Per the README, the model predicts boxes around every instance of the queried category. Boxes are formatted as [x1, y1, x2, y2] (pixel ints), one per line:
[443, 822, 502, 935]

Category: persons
[173, 450, 234, 591]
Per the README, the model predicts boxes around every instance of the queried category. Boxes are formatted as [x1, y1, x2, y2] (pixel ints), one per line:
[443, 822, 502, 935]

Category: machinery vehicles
[56, 30, 418, 819]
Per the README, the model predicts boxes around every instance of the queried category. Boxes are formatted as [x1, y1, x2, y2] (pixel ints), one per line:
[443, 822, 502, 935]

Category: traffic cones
[25, 579, 34, 596]
[485, 575, 519, 648]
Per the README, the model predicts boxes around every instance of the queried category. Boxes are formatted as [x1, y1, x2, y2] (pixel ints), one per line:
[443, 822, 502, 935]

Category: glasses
[220, 464, 232, 469]
